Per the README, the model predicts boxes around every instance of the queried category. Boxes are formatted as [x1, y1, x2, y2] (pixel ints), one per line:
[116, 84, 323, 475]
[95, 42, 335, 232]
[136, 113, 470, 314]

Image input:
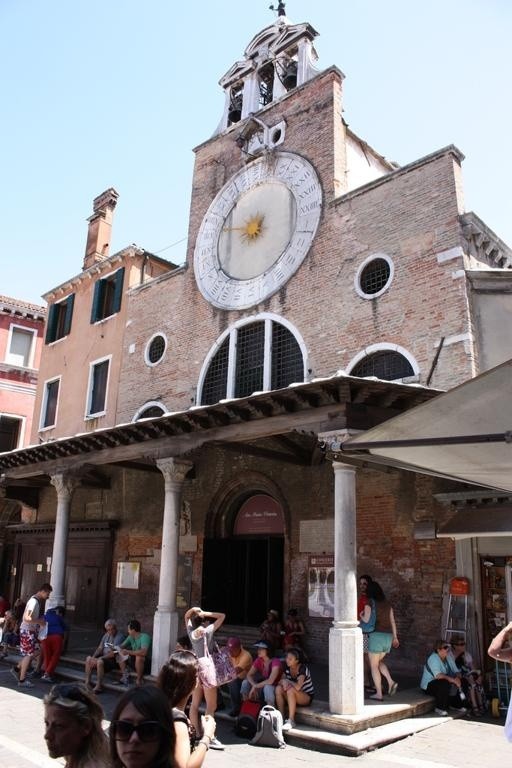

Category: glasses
[109, 720, 161, 742]
[60, 686, 87, 702]
[440, 645, 450, 650]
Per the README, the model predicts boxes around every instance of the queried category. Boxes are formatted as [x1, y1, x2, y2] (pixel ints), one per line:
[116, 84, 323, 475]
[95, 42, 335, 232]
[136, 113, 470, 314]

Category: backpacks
[235, 699, 265, 739]
[249, 705, 287, 749]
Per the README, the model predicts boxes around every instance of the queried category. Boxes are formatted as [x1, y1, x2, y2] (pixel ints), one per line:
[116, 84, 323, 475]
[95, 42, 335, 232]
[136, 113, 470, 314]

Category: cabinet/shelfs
[480, 557, 512, 666]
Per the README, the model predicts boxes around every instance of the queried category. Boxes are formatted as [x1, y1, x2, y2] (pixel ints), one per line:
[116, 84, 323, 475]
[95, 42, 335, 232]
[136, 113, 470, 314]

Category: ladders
[444, 594, 467, 645]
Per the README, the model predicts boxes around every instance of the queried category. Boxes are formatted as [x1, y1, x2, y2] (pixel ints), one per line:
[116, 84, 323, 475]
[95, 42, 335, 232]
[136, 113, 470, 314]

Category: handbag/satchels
[467, 683, 488, 716]
[359, 607, 376, 633]
[197, 648, 238, 688]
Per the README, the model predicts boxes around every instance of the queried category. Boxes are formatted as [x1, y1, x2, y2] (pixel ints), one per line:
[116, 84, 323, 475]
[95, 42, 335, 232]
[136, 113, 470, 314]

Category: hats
[253, 640, 271, 649]
[227, 637, 240, 649]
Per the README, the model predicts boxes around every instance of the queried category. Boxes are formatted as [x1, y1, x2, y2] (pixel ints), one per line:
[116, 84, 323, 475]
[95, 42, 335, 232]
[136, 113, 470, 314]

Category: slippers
[388, 681, 398, 696]
[368, 695, 383, 701]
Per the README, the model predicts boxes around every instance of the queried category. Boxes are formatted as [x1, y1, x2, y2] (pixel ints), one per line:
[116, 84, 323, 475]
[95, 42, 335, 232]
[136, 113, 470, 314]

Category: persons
[283, 608, 305, 656]
[275, 647, 315, 731]
[256, 609, 282, 649]
[357, 573, 383, 693]
[157, 647, 218, 768]
[107, 620, 152, 687]
[2, 599, 26, 653]
[30, 606, 71, 683]
[359, 579, 401, 702]
[216, 637, 252, 717]
[175, 635, 192, 706]
[42, 682, 113, 767]
[109, 686, 181, 768]
[240, 639, 283, 711]
[487, 619, 512, 744]
[185, 607, 225, 749]
[85, 619, 126, 694]
[447, 632, 489, 719]
[10, 583, 52, 688]
[419, 639, 469, 718]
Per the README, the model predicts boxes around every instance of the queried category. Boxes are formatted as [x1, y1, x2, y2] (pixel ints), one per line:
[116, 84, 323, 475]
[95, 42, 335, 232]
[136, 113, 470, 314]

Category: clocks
[192, 152, 323, 311]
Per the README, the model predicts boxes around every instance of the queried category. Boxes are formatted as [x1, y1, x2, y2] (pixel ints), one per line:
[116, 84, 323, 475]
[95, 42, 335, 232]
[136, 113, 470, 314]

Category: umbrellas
[316, 362, 511, 496]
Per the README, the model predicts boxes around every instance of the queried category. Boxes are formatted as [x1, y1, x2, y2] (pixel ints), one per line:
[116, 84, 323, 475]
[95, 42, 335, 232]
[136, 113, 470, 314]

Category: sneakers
[208, 736, 224, 749]
[434, 705, 466, 716]
[283, 719, 296, 730]
[10, 667, 55, 687]
